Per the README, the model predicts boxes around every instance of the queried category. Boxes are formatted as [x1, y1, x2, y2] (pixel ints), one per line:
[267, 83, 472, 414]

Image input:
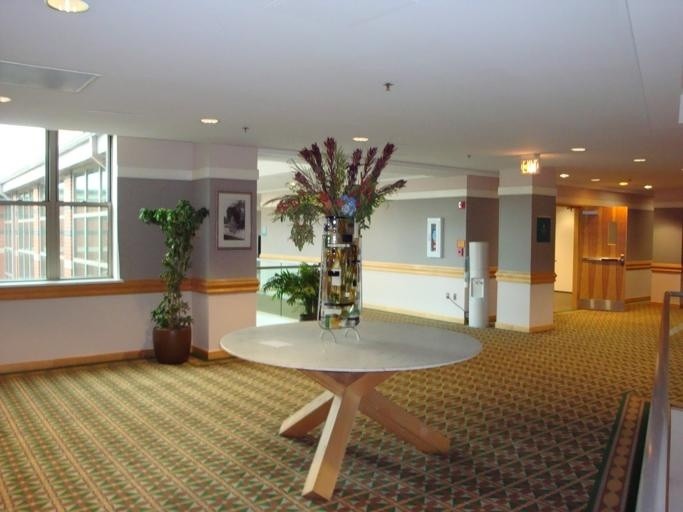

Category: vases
[316, 216, 362, 345]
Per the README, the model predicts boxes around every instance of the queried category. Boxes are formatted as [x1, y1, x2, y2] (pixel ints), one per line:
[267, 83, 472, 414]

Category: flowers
[259, 134, 407, 314]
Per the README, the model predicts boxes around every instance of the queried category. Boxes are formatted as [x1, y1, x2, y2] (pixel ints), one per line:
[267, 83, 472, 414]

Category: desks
[218, 318, 487, 501]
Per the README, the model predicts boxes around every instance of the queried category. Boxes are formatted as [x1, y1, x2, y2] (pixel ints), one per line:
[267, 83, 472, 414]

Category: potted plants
[262, 259, 320, 320]
[136, 197, 208, 366]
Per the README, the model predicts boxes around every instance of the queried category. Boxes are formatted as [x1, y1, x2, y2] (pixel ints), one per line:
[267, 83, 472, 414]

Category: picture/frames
[213, 189, 252, 252]
